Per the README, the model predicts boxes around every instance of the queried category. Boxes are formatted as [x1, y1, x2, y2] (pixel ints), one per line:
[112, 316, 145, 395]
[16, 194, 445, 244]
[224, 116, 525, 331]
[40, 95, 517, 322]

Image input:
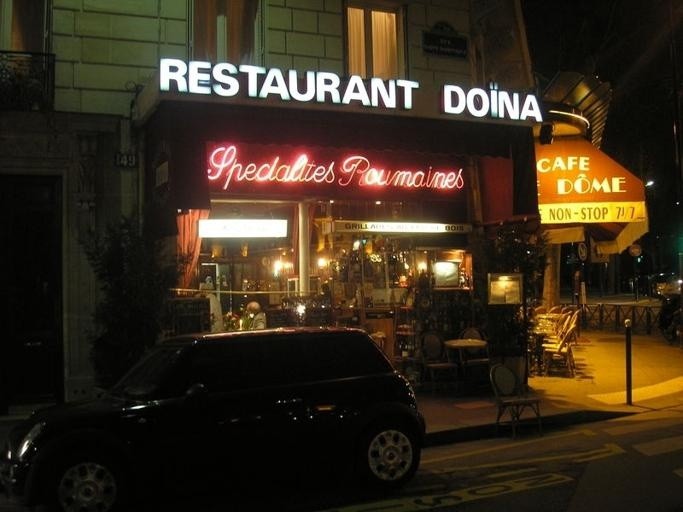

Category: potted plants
[473, 228, 551, 396]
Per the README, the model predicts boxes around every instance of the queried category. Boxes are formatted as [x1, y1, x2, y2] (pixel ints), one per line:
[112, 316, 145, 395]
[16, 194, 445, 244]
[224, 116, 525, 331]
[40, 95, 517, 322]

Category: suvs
[0, 320, 427, 512]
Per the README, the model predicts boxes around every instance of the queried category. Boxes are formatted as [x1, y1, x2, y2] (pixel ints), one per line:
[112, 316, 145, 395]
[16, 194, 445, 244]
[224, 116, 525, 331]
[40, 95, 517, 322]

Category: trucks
[639, 272, 681, 298]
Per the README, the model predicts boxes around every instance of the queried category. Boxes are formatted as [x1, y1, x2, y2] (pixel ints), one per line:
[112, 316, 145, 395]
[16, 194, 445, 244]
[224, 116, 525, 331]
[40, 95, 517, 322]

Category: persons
[198, 282, 223, 333]
[244, 301, 267, 331]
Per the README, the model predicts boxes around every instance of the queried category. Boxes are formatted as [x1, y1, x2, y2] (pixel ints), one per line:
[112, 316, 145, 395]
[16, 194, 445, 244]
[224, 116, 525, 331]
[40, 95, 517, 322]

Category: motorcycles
[656, 293, 682, 345]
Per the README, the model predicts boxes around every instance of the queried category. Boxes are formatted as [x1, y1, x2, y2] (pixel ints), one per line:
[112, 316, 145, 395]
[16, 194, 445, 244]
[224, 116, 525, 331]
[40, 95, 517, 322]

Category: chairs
[519, 300, 579, 377]
[417, 324, 492, 396]
[487, 361, 544, 441]
